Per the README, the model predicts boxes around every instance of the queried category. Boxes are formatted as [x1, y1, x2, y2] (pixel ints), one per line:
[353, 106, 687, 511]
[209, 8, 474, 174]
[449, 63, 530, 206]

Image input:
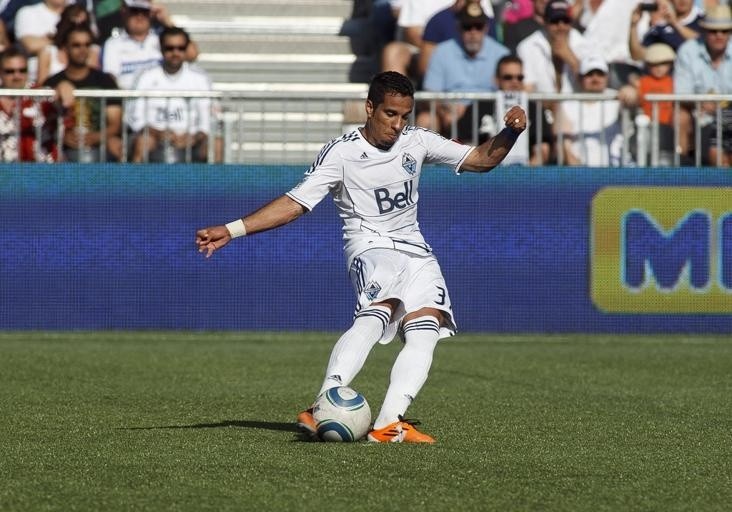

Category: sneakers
[297, 404, 316, 436]
[366, 414, 436, 443]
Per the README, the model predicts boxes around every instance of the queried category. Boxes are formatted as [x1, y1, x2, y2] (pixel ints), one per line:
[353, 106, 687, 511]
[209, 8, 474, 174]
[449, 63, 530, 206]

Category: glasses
[164, 45, 187, 51]
[73, 42, 91, 47]
[5, 68, 26, 73]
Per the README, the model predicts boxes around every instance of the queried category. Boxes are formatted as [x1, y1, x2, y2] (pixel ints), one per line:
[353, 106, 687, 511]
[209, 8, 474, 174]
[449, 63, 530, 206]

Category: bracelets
[225, 219, 246, 239]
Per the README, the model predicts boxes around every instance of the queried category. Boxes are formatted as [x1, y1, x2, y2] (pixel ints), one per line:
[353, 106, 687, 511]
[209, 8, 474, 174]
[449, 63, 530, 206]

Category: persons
[196, 71, 526, 443]
[380, 0, 732, 167]
[0, 0, 223, 165]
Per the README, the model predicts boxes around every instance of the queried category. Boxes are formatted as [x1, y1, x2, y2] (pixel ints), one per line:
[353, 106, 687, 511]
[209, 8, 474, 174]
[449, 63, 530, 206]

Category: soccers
[313, 386, 372, 442]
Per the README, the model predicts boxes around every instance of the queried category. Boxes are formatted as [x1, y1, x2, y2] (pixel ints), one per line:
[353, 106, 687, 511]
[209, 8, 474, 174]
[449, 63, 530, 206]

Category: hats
[459, 0, 732, 81]
